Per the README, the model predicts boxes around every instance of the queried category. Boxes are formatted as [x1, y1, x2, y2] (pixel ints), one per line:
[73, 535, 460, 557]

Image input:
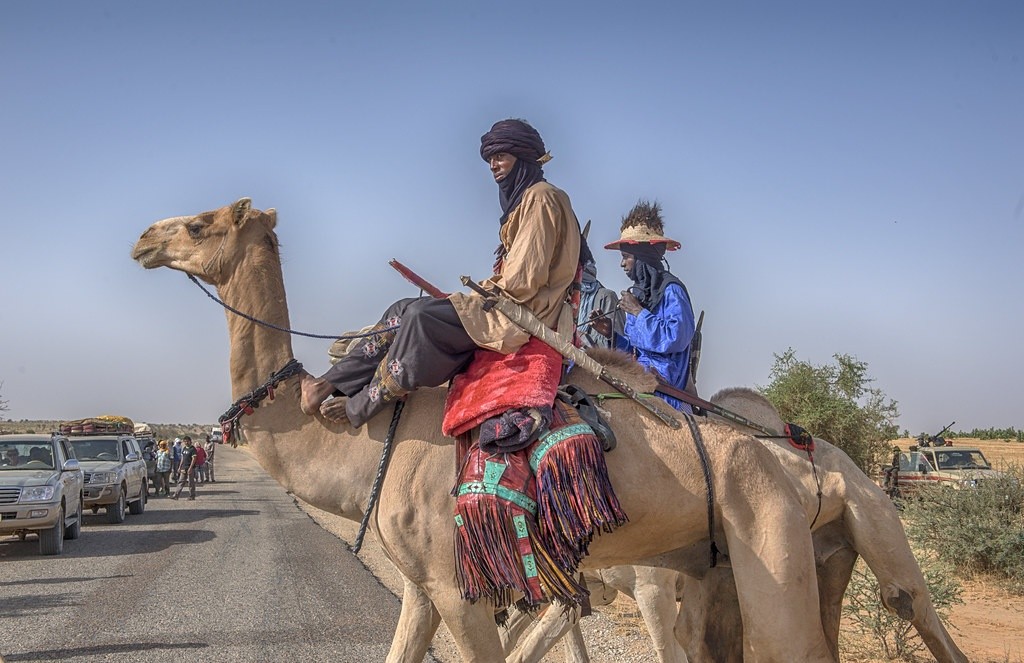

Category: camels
[131, 197, 969, 663]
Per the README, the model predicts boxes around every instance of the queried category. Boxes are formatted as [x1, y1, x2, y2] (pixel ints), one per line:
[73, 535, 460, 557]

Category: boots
[882, 483, 898, 492]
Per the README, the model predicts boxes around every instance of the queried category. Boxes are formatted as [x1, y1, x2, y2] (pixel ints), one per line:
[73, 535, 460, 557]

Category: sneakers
[168, 493, 179, 500]
[185, 496, 195, 500]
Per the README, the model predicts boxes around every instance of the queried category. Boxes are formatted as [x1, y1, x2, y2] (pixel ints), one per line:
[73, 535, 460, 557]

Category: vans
[211, 427, 223, 442]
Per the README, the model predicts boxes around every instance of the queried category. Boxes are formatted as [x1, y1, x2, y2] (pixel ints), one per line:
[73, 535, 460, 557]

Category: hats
[604, 198, 681, 251]
[893, 446, 900, 452]
[480, 119, 553, 169]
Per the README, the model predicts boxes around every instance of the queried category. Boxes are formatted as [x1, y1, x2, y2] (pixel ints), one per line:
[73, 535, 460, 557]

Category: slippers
[556, 384, 617, 452]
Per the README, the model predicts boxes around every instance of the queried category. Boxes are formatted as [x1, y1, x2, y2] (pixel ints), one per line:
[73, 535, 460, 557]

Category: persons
[586, 201, 696, 412]
[144, 433, 217, 499]
[291, 115, 582, 429]
[880, 436, 976, 492]
[4, 448, 22, 469]
[27, 446, 45, 464]
[565, 243, 627, 353]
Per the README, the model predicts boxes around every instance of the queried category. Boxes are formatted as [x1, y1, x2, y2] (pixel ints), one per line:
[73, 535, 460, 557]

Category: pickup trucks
[879, 446, 1020, 511]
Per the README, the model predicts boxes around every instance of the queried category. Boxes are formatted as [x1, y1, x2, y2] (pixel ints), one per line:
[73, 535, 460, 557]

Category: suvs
[0, 434, 82, 556]
[50, 431, 149, 523]
[134, 432, 163, 491]
